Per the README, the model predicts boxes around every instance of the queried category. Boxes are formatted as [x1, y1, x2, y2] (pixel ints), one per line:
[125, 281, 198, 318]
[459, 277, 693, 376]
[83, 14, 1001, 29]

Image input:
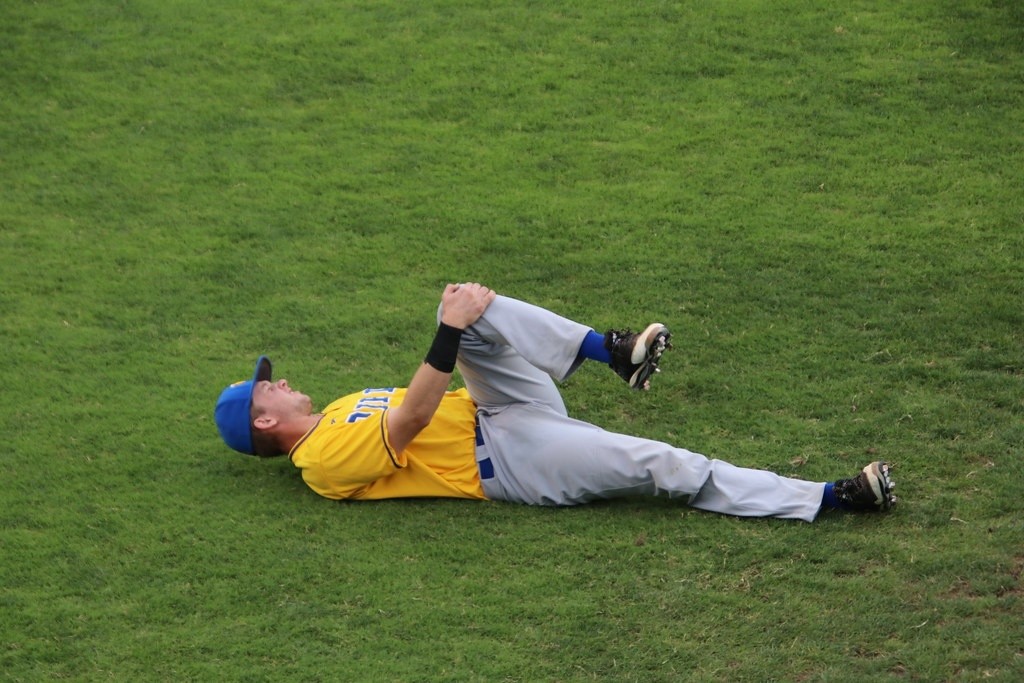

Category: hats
[214, 356, 272, 457]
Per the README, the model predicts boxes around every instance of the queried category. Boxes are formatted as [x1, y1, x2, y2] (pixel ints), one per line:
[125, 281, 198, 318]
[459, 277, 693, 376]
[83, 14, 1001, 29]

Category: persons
[214, 281, 899, 524]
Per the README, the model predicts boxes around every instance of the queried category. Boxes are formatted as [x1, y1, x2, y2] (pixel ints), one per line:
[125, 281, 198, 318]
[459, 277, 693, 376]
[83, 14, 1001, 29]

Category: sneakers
[604, 323, 671, 390]
[832, 461, 896, 512]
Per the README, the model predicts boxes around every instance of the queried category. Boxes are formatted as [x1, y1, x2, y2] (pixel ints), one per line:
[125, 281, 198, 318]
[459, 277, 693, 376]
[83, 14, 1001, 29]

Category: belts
[474, 415, 495, 479]
